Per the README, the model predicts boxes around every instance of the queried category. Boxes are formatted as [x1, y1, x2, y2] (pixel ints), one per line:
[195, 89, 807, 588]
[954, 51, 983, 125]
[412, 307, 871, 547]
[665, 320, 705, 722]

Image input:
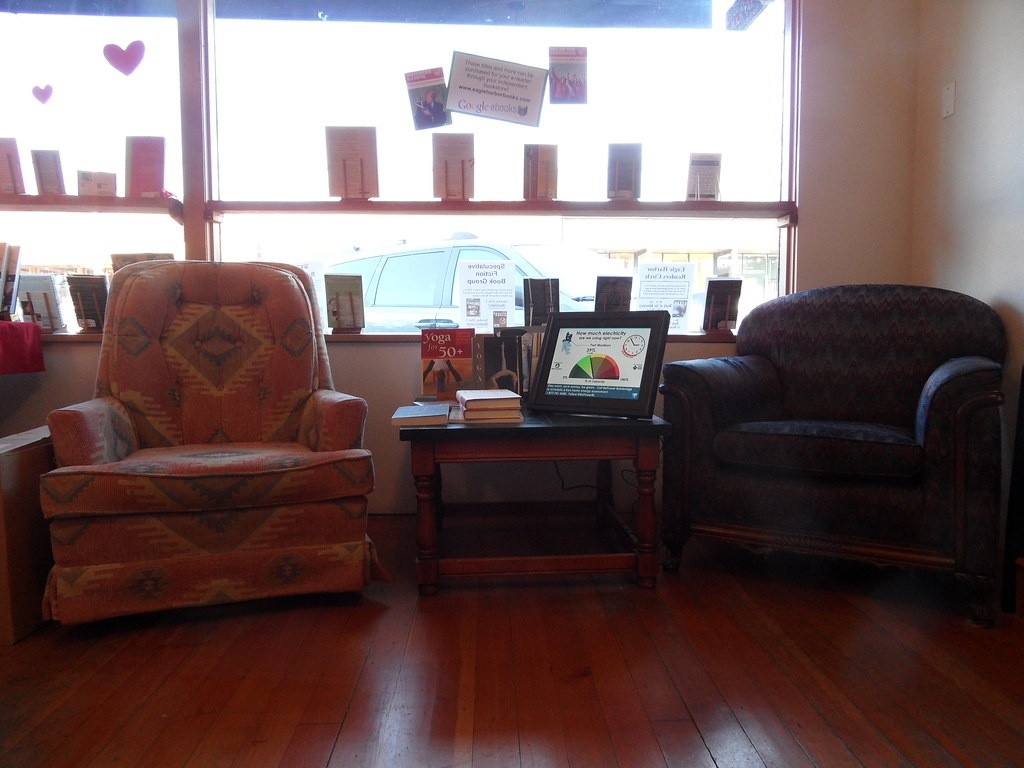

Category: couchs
[658, 283, 1012, 628]
[41, 261, 391, 633]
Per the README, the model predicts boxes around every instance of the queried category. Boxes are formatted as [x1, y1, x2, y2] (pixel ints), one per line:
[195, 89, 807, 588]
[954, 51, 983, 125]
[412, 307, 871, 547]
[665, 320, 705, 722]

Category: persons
[418, 89, 448, 128]
[551, 68, 586, 102]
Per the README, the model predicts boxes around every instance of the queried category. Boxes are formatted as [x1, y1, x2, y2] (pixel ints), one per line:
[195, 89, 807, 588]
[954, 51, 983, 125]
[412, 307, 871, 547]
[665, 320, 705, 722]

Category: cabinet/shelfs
[208, 198, 799, 342]
[0, 194, 182, 343]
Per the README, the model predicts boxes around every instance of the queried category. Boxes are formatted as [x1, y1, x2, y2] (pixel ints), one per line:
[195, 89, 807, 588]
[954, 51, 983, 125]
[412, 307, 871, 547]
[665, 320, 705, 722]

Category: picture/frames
[523, 310, 671, 419]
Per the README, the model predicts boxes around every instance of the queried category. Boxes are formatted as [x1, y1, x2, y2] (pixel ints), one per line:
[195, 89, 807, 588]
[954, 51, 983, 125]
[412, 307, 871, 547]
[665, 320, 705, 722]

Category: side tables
[398, 396, 671, 595]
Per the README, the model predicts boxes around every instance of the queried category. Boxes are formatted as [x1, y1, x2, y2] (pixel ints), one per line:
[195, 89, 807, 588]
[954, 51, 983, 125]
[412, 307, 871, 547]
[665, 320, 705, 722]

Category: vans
[311, 237, 666, 328]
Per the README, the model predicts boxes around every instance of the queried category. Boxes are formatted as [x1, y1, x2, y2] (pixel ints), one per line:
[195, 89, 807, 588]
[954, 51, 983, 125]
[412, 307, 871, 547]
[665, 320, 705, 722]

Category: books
[422, 328, 473, 400]
[687, 153, 721, 200]
[324, 274, 363, 329]
[391, 405, 448, 427]
[0, 139, 26, 195]
[433, 132, 475, 200]
[0, 241, 22, 315]
[523, 145, 558, 199]
[78, 172, 118, 196]
[703, 281, 741, 332]
[15, 274, 61, 329]
[67, 273, 110, 328]
[595, 276, 631, 313]
[31, 150, 66, 195]
[125, 134, 165, 197]
[326, 126, 381, 198]
[521, 331, 548, 394]
[524, 279, 560, 328]
[608, 144, 641, 198]
[449, 387, 525, 424]
